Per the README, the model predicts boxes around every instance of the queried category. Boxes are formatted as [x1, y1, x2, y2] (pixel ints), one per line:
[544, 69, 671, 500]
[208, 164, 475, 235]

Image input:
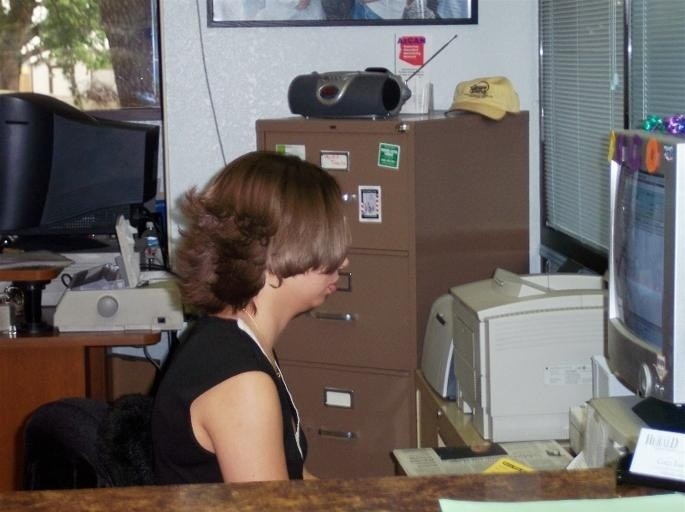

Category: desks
[1, 467, 685, 512]
[411, 367, 483, 446]
[0, 265, 64, 337]
[0, 327, 163, 489]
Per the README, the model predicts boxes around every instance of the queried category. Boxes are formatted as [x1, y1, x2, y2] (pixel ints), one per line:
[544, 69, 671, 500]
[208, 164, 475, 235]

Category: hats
[443, 76, 521, 121]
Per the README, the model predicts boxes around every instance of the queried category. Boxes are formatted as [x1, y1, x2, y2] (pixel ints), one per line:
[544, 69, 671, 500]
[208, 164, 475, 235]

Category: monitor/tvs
[605, 128, 684, 407]
[1, 92, 160, 250]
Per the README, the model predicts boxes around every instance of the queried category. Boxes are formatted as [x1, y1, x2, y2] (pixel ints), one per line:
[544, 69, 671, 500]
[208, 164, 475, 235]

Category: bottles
[142, 221, 159, 267]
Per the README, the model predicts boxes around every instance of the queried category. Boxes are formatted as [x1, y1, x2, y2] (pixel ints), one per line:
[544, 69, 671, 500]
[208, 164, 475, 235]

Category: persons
[142, 150, 349, 484]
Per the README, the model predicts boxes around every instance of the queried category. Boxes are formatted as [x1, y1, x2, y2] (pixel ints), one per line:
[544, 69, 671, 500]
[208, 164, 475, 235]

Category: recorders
[288, 66, 413, 119]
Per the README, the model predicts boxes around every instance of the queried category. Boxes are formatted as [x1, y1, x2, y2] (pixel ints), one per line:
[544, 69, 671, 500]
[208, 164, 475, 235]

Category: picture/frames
[204, 1, 478, 28]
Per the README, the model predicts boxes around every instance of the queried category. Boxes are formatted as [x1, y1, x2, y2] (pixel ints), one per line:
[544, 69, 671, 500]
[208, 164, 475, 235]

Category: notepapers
[645, 136, 660, 173]
[615, 132, 626, 164]
[629, 135, 642, 170]
[607, 128, 616, 164]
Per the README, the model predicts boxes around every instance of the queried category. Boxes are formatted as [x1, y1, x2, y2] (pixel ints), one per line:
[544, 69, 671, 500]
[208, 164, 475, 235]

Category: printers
[54, 214, 184, 333]
[448, 266, 607, 444]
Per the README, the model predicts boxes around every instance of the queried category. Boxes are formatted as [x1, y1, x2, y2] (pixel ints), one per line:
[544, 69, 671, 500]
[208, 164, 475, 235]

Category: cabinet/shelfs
[262, 130, 412, 251]
[274, 254, 412, 373]
[279, 364, 412, 480]
